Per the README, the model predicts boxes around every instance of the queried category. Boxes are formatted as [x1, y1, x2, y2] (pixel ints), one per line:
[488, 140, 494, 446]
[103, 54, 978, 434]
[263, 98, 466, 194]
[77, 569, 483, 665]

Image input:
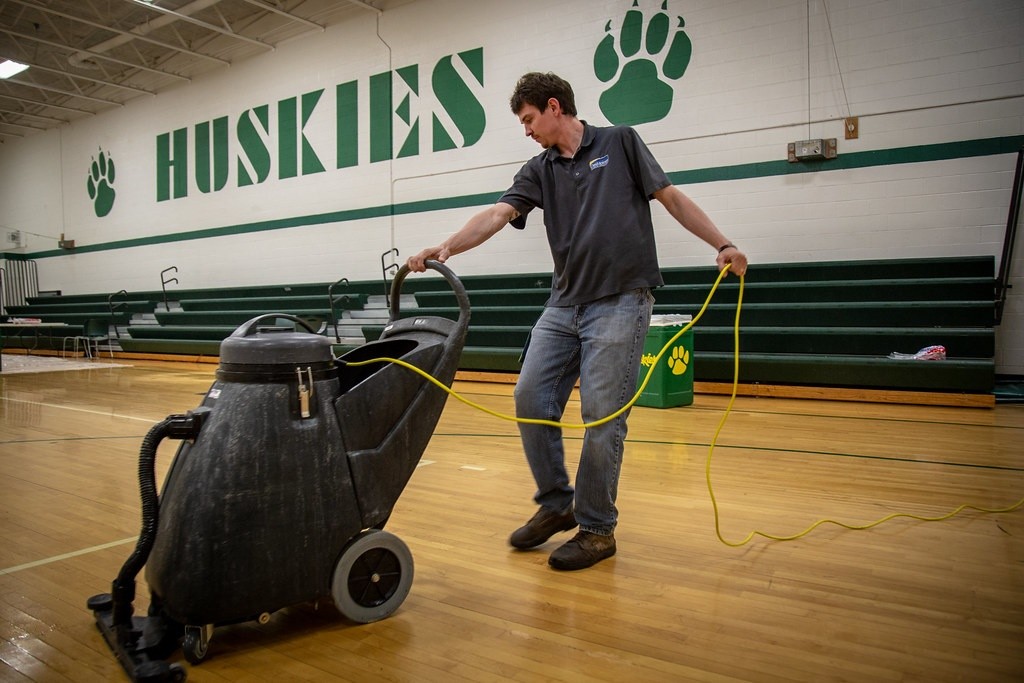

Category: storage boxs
[631, 313, 695, 409]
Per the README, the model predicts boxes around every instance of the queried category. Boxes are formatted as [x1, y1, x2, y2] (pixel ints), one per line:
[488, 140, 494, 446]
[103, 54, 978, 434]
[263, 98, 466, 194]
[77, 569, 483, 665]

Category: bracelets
[718, 242, 737, 253]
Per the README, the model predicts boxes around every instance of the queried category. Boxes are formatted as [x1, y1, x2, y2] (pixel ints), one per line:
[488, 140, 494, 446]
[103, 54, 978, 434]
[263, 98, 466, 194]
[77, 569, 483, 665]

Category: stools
[0, 253, 1002, 409]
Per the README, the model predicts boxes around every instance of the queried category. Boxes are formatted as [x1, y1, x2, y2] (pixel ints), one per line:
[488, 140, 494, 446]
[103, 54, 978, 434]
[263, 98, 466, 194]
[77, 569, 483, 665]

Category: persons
[403, 73, 746, 571]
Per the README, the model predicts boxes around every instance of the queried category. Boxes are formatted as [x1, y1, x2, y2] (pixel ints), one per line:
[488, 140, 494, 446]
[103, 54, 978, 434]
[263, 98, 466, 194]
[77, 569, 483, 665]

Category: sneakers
[548, 529, 617, 571]
[510, 504, 579, 549]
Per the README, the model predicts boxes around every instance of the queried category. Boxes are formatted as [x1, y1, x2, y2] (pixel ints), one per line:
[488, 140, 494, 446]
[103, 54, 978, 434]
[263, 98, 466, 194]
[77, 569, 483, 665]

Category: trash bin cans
[632, 314, 695, 410]
